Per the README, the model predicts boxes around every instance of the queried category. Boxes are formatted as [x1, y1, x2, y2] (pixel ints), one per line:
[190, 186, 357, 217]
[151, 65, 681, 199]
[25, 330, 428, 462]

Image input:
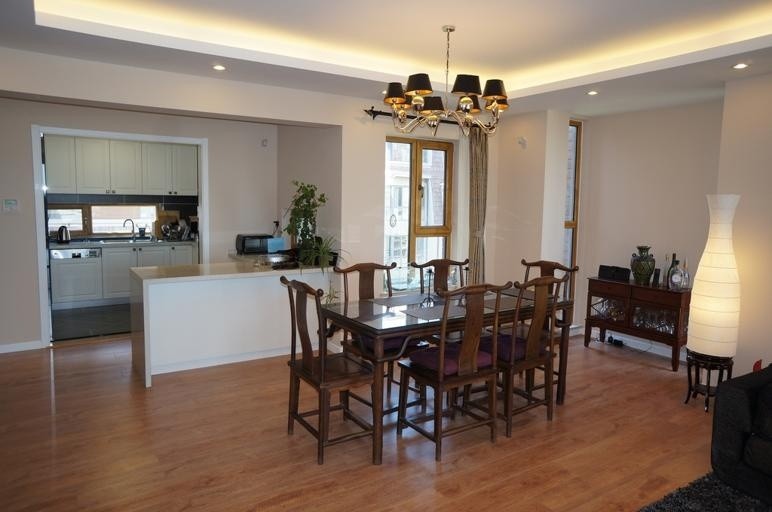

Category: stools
[684, 356, 733, 413]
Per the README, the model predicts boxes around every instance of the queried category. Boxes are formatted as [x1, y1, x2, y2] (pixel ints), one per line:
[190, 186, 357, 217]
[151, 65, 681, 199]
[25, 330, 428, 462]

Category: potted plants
[285, 180, 330, 263]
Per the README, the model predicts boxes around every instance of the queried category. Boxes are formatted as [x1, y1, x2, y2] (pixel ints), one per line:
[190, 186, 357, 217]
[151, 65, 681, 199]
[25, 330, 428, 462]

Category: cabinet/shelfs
[75, 135, 142, 195]
[43, 134, 77, 195]
[169, 246, 192, 266]
[142, 142, 198, 196]
[49, 248, 101, 310]
[102, 246, 169, 307]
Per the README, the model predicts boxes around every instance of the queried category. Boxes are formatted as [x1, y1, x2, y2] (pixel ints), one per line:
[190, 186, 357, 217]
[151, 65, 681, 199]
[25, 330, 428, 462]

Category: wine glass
[642, 305, 674, 335]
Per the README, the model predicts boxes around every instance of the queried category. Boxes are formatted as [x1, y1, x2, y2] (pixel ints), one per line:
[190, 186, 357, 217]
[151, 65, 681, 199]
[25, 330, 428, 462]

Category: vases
[631, 246, 655, 285]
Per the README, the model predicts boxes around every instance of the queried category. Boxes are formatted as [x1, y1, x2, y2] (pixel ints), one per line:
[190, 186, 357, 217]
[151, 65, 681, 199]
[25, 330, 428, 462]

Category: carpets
[640, 467, 766, 510]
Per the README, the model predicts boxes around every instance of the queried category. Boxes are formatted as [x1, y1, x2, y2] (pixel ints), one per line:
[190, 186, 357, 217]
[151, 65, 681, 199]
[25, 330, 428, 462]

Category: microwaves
[235, 232, 273, 255]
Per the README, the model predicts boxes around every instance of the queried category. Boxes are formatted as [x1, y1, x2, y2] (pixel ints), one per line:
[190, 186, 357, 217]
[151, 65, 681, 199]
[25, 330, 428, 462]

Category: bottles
[663, 252, 690, 290]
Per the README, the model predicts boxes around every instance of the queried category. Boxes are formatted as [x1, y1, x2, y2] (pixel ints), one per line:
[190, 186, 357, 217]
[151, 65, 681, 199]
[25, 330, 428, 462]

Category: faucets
[122, 216, 137, 237]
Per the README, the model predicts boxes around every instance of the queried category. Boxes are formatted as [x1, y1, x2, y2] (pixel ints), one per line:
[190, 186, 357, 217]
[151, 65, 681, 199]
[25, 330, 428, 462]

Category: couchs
[711, 364, 768, 500]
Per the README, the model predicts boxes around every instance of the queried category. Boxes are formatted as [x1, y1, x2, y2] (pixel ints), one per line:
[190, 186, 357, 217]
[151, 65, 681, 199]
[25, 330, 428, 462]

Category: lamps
[383, 24, 510, 136]
[684, 195, 741, 361]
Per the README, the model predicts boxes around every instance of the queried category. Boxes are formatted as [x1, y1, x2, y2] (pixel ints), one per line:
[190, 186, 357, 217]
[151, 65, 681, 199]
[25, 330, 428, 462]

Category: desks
[319, 286, 573, 466]
[584, 276, 692, 372]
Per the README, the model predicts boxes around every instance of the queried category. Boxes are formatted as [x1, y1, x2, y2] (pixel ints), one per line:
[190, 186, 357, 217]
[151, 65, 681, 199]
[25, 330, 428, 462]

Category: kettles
[56, 225, 72, 244]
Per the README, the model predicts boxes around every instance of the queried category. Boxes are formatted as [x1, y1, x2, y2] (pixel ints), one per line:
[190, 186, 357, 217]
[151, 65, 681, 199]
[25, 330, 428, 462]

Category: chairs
[487, 259, 578, 406]
[334, 262, 428, 422]
[280, 275, 374, 465]
[397, 281, 513, 462]
[410, 257, 488, 408]
[447, 274, 569, 438]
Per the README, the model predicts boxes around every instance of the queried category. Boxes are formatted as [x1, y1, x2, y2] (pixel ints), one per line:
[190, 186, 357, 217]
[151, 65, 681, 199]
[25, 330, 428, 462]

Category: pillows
[362, 336, 420, 347]
[409, 342, 492, 377]
[480, 333, 529, 361]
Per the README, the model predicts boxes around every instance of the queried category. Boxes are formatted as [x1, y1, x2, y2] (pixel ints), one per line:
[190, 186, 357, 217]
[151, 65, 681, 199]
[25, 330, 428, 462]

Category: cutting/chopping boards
[158, 210, 180, 240]
